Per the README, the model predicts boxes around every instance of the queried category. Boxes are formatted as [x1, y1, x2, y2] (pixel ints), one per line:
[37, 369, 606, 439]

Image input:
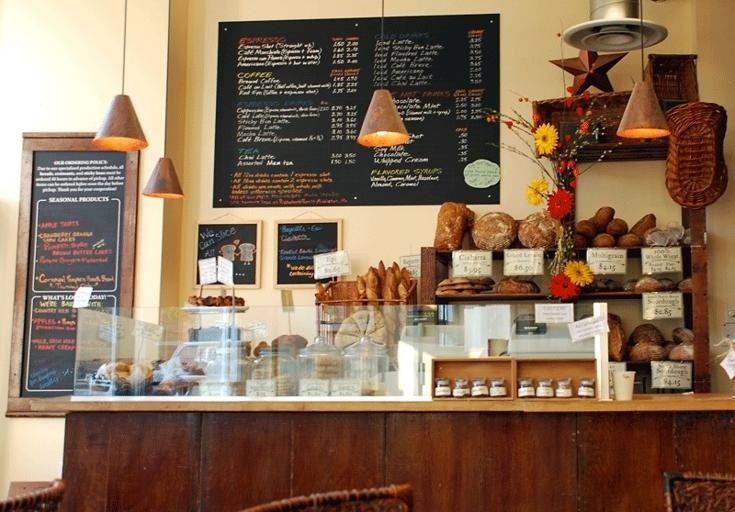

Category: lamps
[141, 1, 184, 199]
[90, 0, 148, 152]
[356, 0, 411, 147]
[617, 0, 671, 139]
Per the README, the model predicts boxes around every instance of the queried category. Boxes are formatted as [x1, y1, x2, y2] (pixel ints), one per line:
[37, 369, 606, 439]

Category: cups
[611, 370, 636, 401]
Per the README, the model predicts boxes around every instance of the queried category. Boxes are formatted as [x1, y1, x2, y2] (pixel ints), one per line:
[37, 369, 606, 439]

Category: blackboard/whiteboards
[213, 14, 501, 206]
[5, 131, 139, 419]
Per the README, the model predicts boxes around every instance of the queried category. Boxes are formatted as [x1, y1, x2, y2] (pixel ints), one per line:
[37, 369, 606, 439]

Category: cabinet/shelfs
[420, 240, 712, 401]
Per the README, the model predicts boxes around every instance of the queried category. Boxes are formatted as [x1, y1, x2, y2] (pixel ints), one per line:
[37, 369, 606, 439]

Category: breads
[623, 277, 695, 291]
[573, 206, 656, 246]
[520, 212, 556, 248]
[332, 310, 387, 349]
[472, 213, 519, 250]
[104, 361, 154, 389]
[355, 260, 410, 304]
[301, 355, 376, 395]
[434, 202, 473, 250]
[605, 314, 696, 360]
[438, 272, 542, 299]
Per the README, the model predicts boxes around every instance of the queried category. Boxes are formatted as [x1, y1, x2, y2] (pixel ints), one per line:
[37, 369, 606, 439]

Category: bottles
[435, 377, 596, 398]
[342, 336, 389, 396]
[297, 336, 341, 396]
[246, 348, 297, 396]
